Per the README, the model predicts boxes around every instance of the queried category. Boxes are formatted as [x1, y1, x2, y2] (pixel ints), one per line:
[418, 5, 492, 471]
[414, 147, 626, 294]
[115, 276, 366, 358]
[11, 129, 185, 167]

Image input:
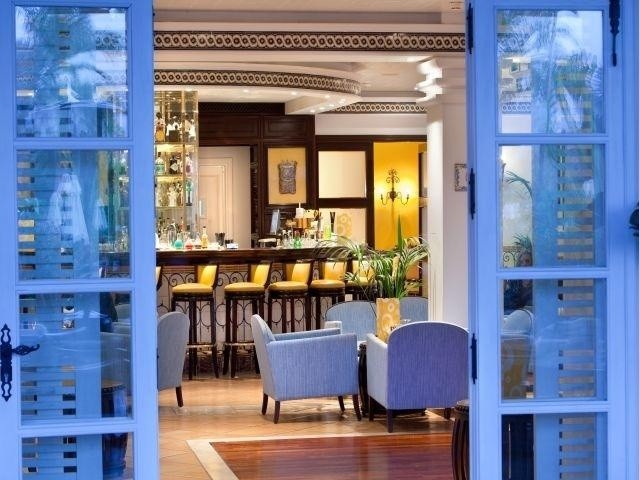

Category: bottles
[155, 151, 193, 176]
[155, 112, 166, 142]
[175, 226, 208, 250]
[276, 220, 331, 248]
[158, 216, 184, 241]
[167, 180, 192, 206]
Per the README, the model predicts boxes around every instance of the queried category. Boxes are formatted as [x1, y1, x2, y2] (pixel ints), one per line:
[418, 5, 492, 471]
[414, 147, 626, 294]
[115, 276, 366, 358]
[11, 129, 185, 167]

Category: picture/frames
[455, 163, 467, 192]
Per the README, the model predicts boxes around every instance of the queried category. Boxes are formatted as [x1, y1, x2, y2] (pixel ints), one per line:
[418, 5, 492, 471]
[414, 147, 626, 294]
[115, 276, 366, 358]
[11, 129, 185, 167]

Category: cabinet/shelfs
[110, 91, 199, 241]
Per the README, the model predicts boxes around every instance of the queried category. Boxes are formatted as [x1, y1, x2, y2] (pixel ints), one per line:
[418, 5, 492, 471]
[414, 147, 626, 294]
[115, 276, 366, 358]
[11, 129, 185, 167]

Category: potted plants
[306, 237, 430, 343]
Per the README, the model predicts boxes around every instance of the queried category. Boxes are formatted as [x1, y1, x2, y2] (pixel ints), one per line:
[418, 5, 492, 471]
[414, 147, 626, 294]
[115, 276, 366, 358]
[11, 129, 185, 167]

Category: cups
[215, 232, 225, 246]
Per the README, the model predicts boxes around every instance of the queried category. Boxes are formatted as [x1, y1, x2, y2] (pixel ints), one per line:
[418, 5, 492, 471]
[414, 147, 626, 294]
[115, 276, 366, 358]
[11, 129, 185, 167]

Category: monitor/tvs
[269, 209, 281, 235]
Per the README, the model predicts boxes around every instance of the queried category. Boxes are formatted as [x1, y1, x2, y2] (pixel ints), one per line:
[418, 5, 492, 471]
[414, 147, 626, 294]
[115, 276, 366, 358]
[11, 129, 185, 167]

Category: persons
[502, 287, 536, 387]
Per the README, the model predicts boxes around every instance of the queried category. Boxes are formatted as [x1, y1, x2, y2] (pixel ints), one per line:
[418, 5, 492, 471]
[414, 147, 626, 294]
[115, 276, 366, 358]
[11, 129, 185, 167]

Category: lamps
[375, 166, 412, 207]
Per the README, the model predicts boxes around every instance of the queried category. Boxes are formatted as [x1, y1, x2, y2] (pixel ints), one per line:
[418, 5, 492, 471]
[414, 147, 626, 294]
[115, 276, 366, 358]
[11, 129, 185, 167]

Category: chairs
[157, 311, 191, 406]
[157, 252, 422, 381]
[250, 296, 469, 432]
